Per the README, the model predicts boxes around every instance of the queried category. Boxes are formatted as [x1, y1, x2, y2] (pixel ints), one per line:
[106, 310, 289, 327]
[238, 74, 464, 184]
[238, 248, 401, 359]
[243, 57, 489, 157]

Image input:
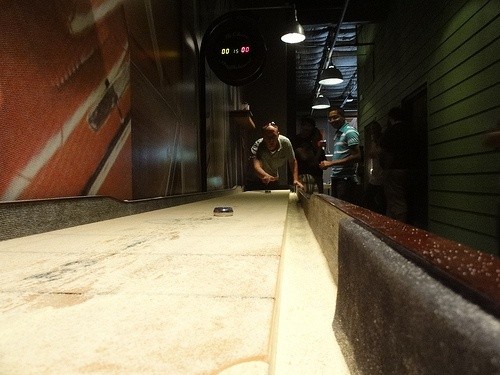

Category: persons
[251, 121, 305, 191]
[294, 107, 415, 222]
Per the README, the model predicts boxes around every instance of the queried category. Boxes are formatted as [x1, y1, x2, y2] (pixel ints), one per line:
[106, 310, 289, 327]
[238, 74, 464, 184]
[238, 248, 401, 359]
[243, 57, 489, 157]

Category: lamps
[318, 56, 344, 85]
[281, 0, 306, 44]
[311, 90, 330, 110]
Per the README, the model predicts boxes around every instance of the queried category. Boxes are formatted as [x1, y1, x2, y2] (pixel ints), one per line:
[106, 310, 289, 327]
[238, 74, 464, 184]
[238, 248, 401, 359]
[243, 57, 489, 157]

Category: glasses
[264, 121, 278, 127]
